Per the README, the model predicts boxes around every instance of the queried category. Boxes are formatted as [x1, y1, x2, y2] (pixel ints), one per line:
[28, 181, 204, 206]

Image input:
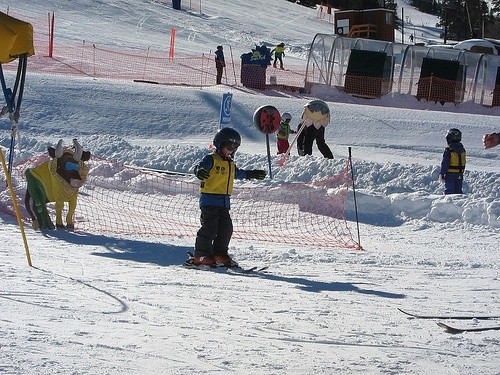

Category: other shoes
[194, 255, 217, 266]
[213, 255, 238, 268]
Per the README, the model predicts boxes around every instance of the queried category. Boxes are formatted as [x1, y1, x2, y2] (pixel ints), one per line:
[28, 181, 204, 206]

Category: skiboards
[437, 322, 500, 334]
[183, 262, 269, 274]
[397, 307, 500, 319]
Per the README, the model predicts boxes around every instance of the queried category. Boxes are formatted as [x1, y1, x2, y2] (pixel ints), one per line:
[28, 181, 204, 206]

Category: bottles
[440, 182, 447, 191]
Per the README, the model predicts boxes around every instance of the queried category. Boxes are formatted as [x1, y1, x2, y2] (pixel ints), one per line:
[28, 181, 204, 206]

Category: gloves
[194, 165, 210, 181]
[246, 170, 266, 180]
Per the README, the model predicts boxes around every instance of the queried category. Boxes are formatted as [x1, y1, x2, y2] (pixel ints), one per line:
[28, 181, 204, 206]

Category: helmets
[213, 128, 241, 149]
[445, 128, 462, 145]
[281, 113, 292, 122]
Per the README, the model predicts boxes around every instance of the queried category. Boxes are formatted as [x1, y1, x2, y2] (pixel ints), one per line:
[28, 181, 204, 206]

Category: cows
[24, 138, 91, 231]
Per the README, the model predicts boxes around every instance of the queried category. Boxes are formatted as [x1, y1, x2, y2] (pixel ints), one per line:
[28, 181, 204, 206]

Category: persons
[483, 132, 500, 147]
[409, 34, 414, 43]
[439, 128, 466, 194]
[277, 113, 297, 155]
[215, 45, 226, 84]
[193, 128, 268, 269]
[271, 42, 286, 70]
